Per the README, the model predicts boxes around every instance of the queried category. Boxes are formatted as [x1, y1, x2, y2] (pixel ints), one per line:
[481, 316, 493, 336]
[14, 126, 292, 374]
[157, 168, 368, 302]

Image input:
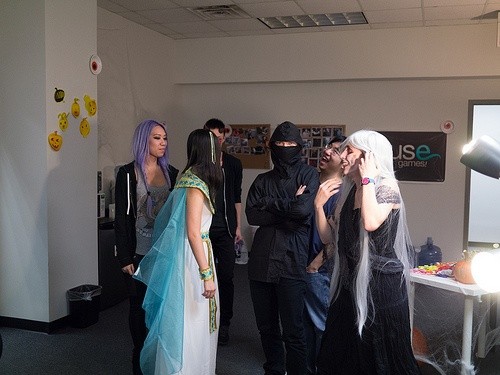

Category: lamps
[460, 133, 500, 180]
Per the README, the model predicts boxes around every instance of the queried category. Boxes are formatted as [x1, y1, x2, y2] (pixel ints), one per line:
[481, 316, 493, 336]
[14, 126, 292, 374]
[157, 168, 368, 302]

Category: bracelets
[200, 267, 213, 280]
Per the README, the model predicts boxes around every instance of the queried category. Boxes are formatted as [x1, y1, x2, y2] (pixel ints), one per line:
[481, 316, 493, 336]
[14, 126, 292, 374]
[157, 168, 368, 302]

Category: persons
[301, 136, 347, 375]
[132, 129, 223, 375]
[115, 120, 179, 375]
[314, 129, 419, 375]
[203, 118, 244, 344]
[244, 122, 320, 375]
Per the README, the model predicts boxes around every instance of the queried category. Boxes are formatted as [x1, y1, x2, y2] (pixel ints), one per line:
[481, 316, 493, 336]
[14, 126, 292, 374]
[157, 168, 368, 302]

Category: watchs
[360, 177, 374, 186]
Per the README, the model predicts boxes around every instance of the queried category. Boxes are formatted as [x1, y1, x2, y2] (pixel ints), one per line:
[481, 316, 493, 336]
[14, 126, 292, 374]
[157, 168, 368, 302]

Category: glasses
[324, 143, 341, 157]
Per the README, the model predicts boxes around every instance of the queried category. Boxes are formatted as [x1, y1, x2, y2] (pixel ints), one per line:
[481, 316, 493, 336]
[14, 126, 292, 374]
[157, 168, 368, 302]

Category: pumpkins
[453, 249, 478, 283]
[412, 327, 428, 369]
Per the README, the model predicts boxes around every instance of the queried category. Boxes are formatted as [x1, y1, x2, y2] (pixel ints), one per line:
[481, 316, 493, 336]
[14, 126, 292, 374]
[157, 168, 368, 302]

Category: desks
[407, 268, 500, 375]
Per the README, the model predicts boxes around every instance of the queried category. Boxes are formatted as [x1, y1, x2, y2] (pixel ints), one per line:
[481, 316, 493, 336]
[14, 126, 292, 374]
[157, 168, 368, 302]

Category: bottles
[419, 236, 442, 266]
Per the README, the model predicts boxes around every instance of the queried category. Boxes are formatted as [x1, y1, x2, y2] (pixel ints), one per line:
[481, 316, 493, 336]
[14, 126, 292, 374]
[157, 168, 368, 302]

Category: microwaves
[97, 193, 105, 218]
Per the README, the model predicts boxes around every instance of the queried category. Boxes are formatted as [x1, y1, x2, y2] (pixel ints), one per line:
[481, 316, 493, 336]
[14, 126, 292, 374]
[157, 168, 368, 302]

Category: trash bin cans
[67, 284, 102, 329]
